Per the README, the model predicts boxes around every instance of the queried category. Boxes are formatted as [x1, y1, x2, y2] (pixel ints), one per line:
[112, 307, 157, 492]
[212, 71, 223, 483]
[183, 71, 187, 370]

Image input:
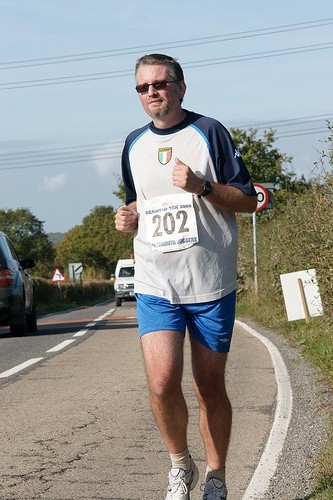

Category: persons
[114, 53, 258, 499]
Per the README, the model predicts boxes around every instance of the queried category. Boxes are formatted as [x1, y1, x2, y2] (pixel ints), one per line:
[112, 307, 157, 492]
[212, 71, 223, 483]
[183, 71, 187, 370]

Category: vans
[110, 258, 135, 307]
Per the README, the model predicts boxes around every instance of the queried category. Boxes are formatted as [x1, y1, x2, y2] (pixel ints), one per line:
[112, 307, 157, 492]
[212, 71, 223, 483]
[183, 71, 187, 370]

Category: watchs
[197, 180, 212, 199]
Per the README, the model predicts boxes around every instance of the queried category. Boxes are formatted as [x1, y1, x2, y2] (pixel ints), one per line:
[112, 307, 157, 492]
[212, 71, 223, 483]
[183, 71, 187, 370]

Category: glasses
[135, 80, 178, 93]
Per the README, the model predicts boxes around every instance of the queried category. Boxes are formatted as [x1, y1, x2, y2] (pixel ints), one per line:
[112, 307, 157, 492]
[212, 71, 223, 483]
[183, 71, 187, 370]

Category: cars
[0, 231, 38, 335]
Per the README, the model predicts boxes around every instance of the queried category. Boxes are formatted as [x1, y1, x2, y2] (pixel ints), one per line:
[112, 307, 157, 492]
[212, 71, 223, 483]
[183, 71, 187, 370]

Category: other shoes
[199, 478, 228, 500]
[164, 455, 199, 500]
